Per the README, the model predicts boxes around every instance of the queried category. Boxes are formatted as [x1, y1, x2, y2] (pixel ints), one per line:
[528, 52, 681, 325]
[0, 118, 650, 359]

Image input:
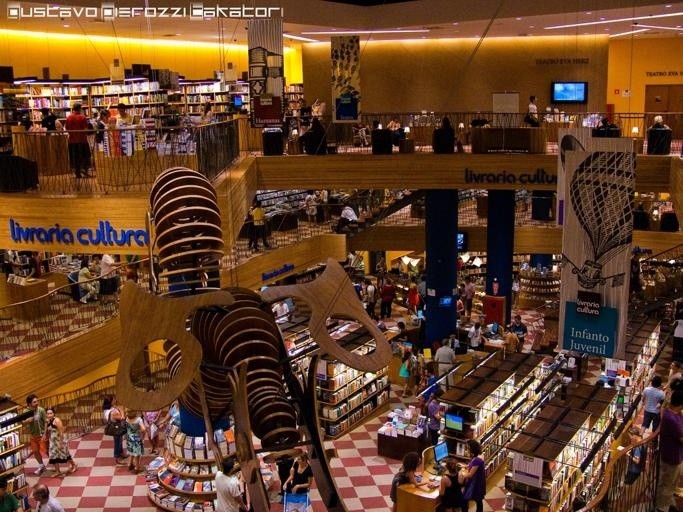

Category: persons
[602, 118, 609, 128]
[34, 485, 65, 511]
[102, 389, 180, 472]
[22, 394, 51, 474]
[652, 116, 670, 128]
[248, 188, 683, 512]
[213, 455, 245, 510]
[42, 410, 76, 479]
[471, 112, 488, 127]
[19, 104, 134, 179]
[0, 476, 19, 510]
[528, 95, 538, 126]
[386, 117, 401, 132]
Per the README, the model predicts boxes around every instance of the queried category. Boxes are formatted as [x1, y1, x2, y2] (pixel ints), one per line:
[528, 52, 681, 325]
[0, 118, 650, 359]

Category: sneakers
[33, 465, 77, 478]
[115, 454, 144, 472]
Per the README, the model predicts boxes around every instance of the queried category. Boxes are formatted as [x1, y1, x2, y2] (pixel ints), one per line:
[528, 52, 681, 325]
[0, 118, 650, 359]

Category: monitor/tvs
[455, 232, 467, 252]
[445, 413, 464, 432]
[433, 441, 449, 469]
[551, 82, 588, 104]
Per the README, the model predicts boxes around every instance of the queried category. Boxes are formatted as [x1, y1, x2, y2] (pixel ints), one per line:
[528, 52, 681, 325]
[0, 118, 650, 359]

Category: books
[285, 86, 304, 115]
[0, 82, 251, 155]
[3, 248, 120, 304]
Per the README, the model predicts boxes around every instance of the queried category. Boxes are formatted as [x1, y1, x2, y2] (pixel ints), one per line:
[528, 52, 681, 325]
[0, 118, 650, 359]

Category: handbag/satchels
[104, 420, 127, 436]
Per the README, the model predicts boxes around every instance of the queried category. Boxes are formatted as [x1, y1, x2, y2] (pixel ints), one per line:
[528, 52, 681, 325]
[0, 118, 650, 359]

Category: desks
[396, 463, 467, 512]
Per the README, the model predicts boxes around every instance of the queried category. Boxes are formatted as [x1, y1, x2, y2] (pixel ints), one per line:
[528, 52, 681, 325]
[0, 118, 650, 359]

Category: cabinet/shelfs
[306, 325, 390, 439]
[0, 397, 34, 512]
[146, 419, 271, 512]
[437, 349, 547, 482]
[278, 316, 320, 386]
[420, 317, 505, 352]
[518, 270, 561, 308]
[503, 380, 639, 512]
[603, 316, 662, 384]
